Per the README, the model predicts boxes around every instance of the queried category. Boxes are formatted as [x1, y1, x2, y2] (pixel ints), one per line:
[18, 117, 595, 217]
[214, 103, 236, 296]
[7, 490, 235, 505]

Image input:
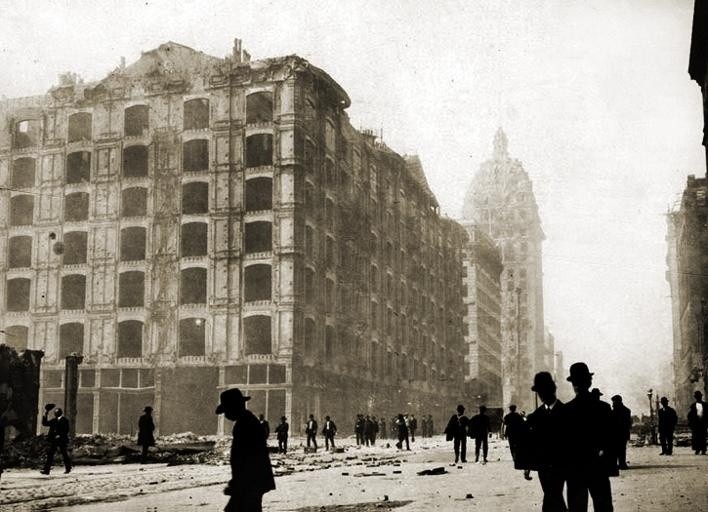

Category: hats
[455, 404, 465, 410]
[694, 391, 702, 398]
[531, 370, 555, 391]
[661, 396, 668, 403]
[279, 416, 287, 421]
[215, 387, 250, 414]
[565, 362, 594, 381]
[592, 388, 603, 396]
[46, 403, 54, 410]
[144, 406, 153, 411]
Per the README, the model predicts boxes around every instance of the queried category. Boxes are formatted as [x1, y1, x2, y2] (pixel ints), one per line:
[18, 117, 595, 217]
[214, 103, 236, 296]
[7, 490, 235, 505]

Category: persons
[42, 403, 74, 474]
[687, 391, 708, 455]
[322, 415, 337, 451]
[258, 413, 269, 444]
[425, 414, 434, 437]
[0, 402, 15, 476]
[469, 406, 492, 462]
[138, 406, 155, 464]
[275, 415, 289, 454]
[590, 388, 602, 399]
[520, 410, 526, 418]
[354, 412, 417, 450]
[306, 414, 318, 448]
[502, 405, 525, 458]
[421, 415, 427, 438]
[610, 394, 631, 470]
[521, 372, 572, 511]
[214, 389, 276, 511]
[446, 405, 472, 462]
[544, 361, 623, 511]
[658, 396, 678, 455]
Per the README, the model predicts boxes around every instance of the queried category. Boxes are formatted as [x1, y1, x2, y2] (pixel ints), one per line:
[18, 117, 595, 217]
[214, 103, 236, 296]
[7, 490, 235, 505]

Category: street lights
[646, 388, 655, 444]
[514, 286, 522, 403]
